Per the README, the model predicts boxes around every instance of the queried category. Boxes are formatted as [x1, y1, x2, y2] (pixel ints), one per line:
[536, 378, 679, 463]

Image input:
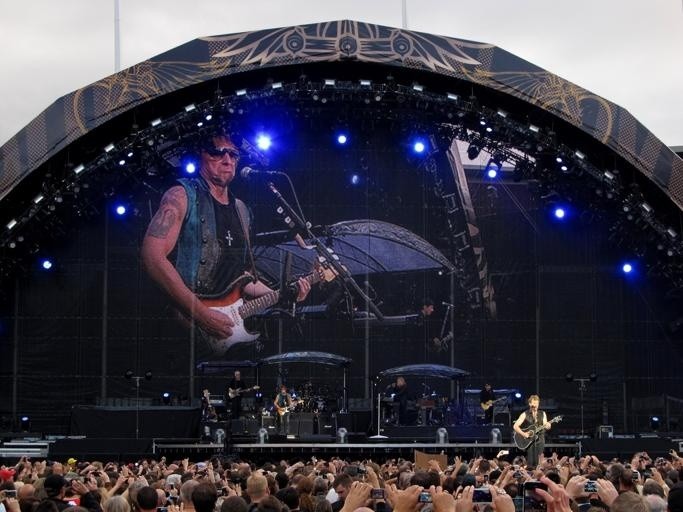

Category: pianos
[377, 396, 401, 406]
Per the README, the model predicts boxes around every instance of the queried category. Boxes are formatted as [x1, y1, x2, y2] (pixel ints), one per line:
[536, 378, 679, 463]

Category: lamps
[426, 92, 553, 201]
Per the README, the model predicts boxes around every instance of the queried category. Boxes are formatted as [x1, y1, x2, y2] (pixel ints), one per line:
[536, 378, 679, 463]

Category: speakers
[232, 419, 243, 435]
[317, 416, 338, 442]
[299, 412, 315, 436]
[288, 413, 299, 436]
[261, 415, 275, 433]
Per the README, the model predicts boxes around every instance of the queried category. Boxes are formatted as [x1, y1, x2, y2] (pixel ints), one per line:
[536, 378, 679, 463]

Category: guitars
[426, 332, 454, 353]
[161, 265, 348, 376]
[203, 389, 218, 420]
[229, 386, 260, 398]
[278, 400, 304, 416]
[481, 396, 507, 410]
[514, 415, 566, 450]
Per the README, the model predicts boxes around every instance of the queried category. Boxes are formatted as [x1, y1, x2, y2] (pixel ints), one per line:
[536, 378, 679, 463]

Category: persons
[2, 449, 683, 512]
[394, 376, 407, 425]
[396, 297, 440, 349]
[481, 383, 496, 418]
[514, 396, 552, 469]
[139, 129, 313, 340]
[200, 369, 294, 416]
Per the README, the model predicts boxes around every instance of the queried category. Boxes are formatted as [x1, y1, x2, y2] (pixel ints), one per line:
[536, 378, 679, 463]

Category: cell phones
[484, 474, 488, 483]
[5, 490, 17, 499]
[523, 481, 548, 512]
[512, 497, 523, 512]
[667, 449, 672, 455]
[643, 469, 653, 476]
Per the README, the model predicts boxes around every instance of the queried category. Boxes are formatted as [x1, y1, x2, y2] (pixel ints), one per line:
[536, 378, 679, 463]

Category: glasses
[201, 146, 240, 160]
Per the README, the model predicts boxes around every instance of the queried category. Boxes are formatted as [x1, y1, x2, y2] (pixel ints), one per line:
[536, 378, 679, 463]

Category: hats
[0, 457, 78, 495]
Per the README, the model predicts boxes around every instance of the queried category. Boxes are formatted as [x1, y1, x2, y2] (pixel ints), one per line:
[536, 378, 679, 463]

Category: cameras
[418, 491, 433, 503]
[371, 488, 384, 499]
[583, 481, 598, 493]
[216, 487, 228, 496]
[472, 488, 492, 504]
[157, 507, 167, 512]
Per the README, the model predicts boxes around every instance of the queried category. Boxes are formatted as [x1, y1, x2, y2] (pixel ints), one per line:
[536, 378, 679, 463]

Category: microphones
[531, 406, 536, 416]
[442, 301, 456, 308]
[240, 167, 276, 183]
